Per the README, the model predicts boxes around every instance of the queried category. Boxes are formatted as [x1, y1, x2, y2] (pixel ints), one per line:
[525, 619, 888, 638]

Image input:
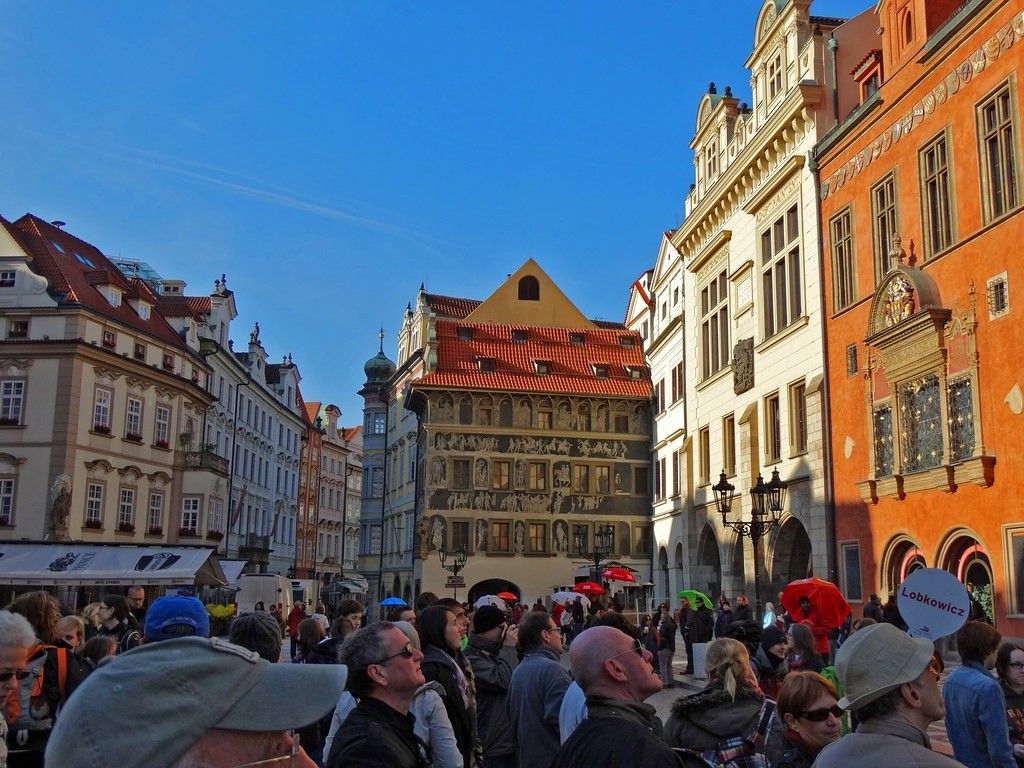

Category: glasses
[376, 644, 413, 664]
[270, 609, 275, 611]
[548, 628, 561, 633]
[234, 734, 300, 768]
[926, 650, 944, 673]
[610, 639, 642, 659]
[695, 602, 700, 603]
[799, 705, 844, 722]
[99, 607, 108, 611]
[130, 597, 144, 602]
[660, 606, 666, 608]
[60, 635, 77, 641]
[1007, 663, 1024, 669]
[0, 671, 31, 680]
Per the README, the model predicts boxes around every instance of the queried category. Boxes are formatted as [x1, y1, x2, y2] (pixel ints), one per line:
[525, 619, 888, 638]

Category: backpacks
[671, 698, 777, 768]
[2, 643, 51, 731]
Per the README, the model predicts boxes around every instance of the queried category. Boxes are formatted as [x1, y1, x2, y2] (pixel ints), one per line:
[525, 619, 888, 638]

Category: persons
[0, 586, 1024, 768]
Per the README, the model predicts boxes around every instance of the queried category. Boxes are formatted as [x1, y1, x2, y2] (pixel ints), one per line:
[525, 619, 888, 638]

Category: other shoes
[679, 670, 694, 674]
[663, 684, 674, 688]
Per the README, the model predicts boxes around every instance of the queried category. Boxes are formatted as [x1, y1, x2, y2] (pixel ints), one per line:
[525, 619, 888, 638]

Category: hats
[762, 625, 787, 649]
[575, 596, 582, 599]
[473, 606, 505, 634]
[295, 601, 303, 605]
[104, 595, 129, 618]
[44, 595, 347, 768]
[835, 623, 935, 711]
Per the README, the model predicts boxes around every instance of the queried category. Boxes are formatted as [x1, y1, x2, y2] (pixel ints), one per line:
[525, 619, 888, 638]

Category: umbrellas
[551, 592, 591, 608]
[497, 591, 517, 600]
[473, 595, 506, 612]
[781, 577, 851, 629]
[379, 597, 407, 606]
[571, 581, 605, 595]
[601, 567, 636, 583]
[678, 591, 713, 610]
